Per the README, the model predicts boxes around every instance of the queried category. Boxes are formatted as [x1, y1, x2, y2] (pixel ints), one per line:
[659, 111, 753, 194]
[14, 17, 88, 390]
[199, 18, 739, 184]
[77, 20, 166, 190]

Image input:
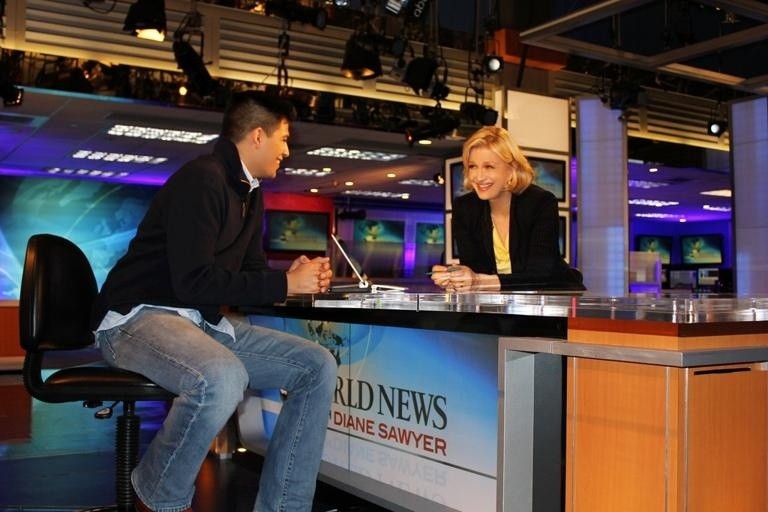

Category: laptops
[331, 233, 408, 291]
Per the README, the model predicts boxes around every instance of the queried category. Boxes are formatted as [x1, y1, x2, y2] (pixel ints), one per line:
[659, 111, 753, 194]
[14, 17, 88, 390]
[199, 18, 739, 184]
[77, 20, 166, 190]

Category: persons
[431, 126, 589, 292]
[87, 89, 339, 511]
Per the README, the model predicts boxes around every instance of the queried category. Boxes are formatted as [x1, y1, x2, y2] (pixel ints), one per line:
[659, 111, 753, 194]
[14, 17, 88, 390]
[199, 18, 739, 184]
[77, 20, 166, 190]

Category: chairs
[20, 234, 179, 512]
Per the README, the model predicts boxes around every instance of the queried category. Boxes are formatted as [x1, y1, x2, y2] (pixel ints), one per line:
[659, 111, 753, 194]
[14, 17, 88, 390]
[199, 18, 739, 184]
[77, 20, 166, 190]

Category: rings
[461, 280, 464, 287]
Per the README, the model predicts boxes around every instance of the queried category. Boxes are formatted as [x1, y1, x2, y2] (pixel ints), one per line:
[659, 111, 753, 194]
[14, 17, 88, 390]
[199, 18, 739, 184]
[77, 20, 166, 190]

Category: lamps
[400, 51, 452, 102]
[172, 10, 222, 97]
[366, 30, 408, 60]
[123, 2, 168, 43]
[407, 113, 458, 147]
[339, 31, 384, 82]
[466, 52, 506, 93]
[460, 102, 498, 127]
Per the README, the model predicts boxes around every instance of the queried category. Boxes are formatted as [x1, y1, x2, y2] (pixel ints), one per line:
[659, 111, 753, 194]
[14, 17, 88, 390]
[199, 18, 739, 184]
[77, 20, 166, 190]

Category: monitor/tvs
[680, 233, 724, 266]
[635, 234, 672, 264]
[264, 209, 330, 253]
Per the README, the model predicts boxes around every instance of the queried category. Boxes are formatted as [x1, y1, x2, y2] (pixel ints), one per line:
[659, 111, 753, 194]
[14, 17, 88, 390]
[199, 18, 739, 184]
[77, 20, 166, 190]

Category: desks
[212, 290, 768, 512]
